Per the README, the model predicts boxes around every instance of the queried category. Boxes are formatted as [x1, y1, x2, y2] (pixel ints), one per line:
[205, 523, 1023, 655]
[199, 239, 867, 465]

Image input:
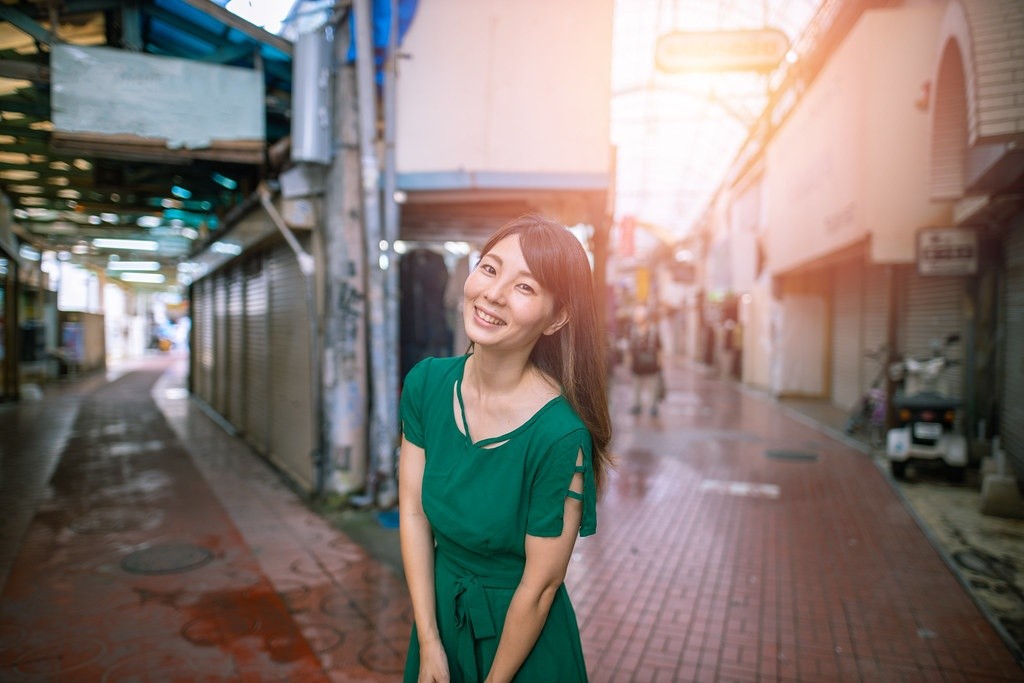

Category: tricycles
[883, 333, 970, 481]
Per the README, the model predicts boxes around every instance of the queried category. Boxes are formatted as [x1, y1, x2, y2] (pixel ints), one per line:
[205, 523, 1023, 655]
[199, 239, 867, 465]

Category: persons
[398, 220, 614, 683]
[716, 319, 735, 378]
[62, 314, 85, 376]
[628, 309, 662, 416]
